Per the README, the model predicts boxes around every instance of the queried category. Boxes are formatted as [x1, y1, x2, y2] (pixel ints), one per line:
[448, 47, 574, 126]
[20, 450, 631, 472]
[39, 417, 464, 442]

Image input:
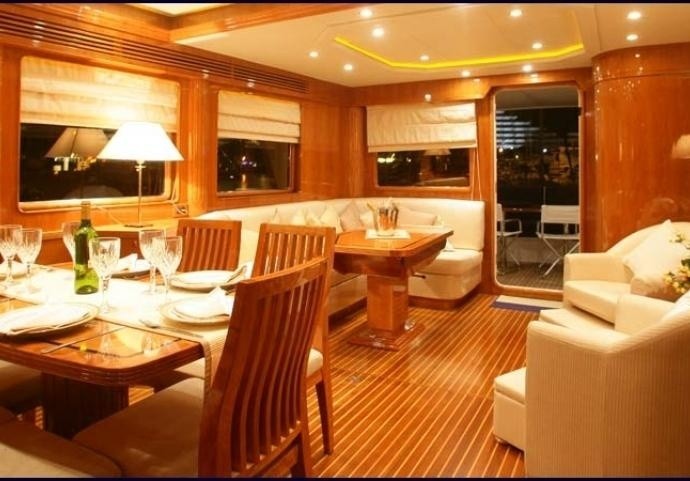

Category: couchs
[187, 193, 489, 331]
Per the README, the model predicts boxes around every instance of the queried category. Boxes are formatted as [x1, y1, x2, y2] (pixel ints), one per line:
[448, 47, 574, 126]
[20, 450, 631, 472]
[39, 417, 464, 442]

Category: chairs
[496, 201, 526, 279]
[249, 222, 336, 454]
[175, 219, 242, 272]
[492, 287, 690, 480]
[1, 406, 121, 477]
[539, 216, 689, 331]
[72, 255, 327, 477]
[534, 201, 580, 277]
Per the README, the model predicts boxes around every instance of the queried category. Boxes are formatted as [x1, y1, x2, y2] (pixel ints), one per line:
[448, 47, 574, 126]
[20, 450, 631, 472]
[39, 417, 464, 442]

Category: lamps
[43, 127, 110, 198]
[97, 120, 189, 227]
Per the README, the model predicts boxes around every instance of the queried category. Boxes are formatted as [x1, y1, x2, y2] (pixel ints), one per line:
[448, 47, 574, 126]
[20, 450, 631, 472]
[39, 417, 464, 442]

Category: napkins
[115, 253, 149, 271]
[3, 303, 90, 335]
[175, 286, 235, 319]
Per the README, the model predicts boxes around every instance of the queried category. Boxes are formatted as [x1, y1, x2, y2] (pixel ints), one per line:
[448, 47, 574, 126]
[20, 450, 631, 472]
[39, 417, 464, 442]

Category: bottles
[75, 200, 100, 296]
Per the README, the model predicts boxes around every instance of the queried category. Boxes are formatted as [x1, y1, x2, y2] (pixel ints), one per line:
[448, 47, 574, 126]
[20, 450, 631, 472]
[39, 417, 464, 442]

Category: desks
[326, 228, 455, 355]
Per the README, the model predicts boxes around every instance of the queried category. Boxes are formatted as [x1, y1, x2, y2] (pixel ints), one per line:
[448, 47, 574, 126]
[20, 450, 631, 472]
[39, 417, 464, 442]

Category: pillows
[620, 219, 690, 283]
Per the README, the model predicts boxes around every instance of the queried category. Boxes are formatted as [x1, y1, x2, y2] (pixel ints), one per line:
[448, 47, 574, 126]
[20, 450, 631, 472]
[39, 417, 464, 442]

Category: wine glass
[0, 224, 24, 286]
[139, 227, 183, 300]
[88, 236, 121, 313]
[12, 227, 43, 295]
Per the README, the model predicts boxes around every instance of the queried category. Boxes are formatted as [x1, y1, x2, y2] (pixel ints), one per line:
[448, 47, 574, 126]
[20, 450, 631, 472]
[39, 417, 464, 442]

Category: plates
[0, 301, 98, 340]
[158, 296, 230, 325]
[110, 256, 153, 279]
[169, 268, 246, 291]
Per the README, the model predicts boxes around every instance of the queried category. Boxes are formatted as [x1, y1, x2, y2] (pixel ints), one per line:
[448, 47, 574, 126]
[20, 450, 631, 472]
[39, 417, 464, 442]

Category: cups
[372, 207, 399, 237]
[62, 220, 83, 260]
